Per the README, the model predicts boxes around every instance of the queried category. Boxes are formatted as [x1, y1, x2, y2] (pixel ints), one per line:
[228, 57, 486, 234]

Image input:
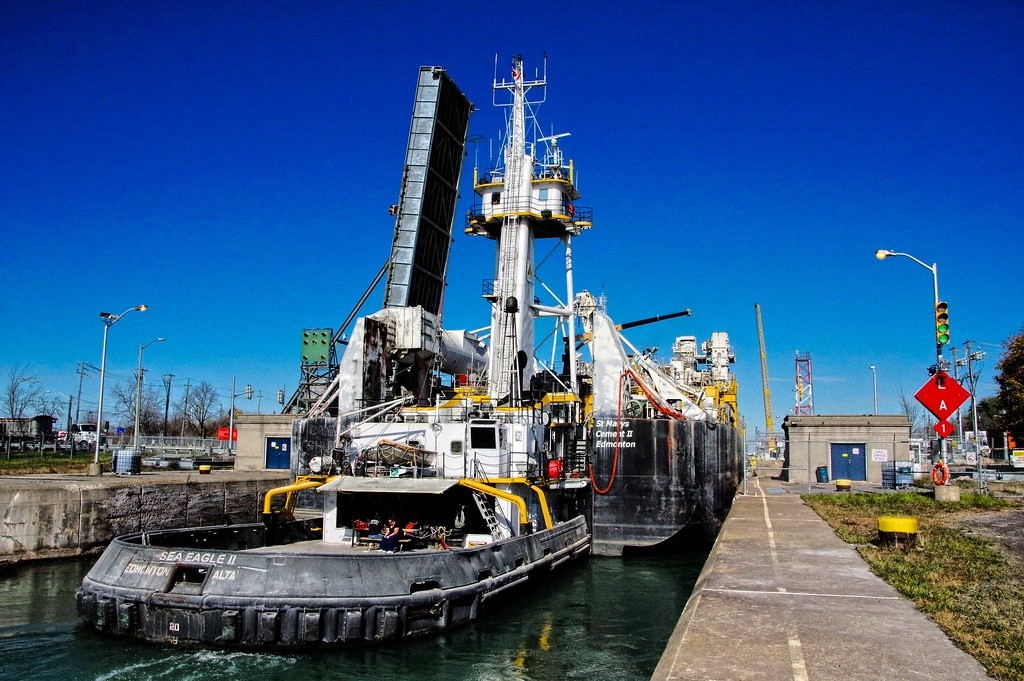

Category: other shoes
[378, 549, 394, 555]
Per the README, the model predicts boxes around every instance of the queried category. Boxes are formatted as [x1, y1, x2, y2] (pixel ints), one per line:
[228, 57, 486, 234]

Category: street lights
[874, 249, 960, 502]
[86, 305, 147, 477]
[134, 338, 166, 451]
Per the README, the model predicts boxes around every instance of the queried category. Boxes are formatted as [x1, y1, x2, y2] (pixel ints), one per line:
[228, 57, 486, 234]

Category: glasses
[389, 521, 393, 523]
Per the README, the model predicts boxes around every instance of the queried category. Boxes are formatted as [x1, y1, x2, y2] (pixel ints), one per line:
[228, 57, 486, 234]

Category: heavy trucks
[1, 415, 108, 452]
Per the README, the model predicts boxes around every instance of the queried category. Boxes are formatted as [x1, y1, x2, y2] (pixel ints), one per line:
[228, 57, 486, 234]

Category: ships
[284, 49, 747, 558]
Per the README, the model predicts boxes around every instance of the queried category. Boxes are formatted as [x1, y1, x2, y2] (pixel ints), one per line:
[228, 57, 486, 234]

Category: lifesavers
[933, 463, 948, 486]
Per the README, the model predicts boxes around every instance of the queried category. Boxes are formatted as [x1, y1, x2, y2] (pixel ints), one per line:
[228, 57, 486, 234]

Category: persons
[378, 516, 404, 554]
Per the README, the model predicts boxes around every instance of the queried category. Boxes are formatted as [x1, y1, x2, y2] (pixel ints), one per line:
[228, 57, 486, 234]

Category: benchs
[359, 537, 411, 553]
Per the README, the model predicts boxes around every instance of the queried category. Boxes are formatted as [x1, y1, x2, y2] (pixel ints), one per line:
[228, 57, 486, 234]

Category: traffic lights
[935, 301, 950, 346]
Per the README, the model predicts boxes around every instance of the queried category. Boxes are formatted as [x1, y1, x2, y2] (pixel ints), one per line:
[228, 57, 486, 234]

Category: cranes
[755, 303, 779, 459]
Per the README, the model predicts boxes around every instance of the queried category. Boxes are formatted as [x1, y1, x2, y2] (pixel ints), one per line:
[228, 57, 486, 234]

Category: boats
[72, 393, 592, 648]
